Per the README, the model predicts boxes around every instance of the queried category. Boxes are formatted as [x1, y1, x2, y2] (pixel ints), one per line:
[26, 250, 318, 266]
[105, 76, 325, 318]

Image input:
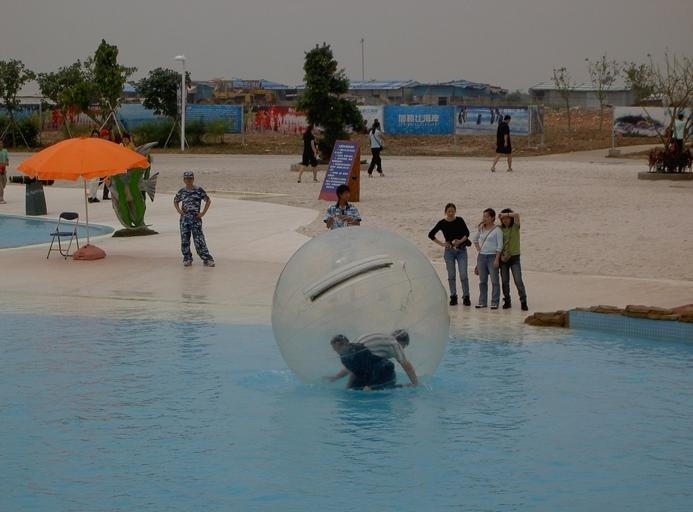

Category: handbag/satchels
[380, 145, 383, 151]
[475, 265, 490, 276]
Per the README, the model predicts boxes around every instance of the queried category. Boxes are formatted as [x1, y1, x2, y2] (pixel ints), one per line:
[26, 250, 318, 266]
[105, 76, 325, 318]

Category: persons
[323, 185, 361, 230]
[352, 329, 419, 385]
[324, 335, 395, 390]
[458, 106, 503, 124]
[428, 204, 528, 311]
[0, 138, 8, 204]
[121, 134, 134, 151]
[174, 171, 215, 267]
[491, 115, 513, 172]
[88, 129, 100, 202]
[297, 125, 321, 183]
[99, 129, 112, 200]
[368, 122, 385, 176]
[671, 114, 686, 139]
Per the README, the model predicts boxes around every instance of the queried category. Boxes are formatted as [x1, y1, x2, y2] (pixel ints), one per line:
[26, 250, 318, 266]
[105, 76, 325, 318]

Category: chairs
[46, 212, 79, 260]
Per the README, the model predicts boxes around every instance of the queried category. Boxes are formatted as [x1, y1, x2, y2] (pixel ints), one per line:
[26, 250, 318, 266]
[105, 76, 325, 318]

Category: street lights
[174, 53, 187, 153]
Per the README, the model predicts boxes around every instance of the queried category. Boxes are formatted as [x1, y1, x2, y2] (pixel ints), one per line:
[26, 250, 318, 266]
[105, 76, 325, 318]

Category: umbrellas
[17, 136, 151, 260]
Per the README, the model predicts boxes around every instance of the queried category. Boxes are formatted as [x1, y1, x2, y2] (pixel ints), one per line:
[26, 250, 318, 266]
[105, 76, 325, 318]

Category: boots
[463, 295, 471, 305]
[450, 295, 457, 305]
[502, 297, 511, 309]
[519, 296, 528, 310]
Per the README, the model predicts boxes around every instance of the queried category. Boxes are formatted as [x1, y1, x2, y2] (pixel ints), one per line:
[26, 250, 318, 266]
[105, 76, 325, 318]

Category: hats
[184, 171, 194, 180]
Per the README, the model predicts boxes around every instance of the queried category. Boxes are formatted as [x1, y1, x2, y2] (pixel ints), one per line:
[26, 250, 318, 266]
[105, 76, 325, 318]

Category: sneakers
[490, 304, 499, 309]
[203, 260, 215, 267]
[93, 197, 101, 203]
[183, 260, 193, 266]
[88, 197, 93, 203]
[475, 304, 488, 308]
